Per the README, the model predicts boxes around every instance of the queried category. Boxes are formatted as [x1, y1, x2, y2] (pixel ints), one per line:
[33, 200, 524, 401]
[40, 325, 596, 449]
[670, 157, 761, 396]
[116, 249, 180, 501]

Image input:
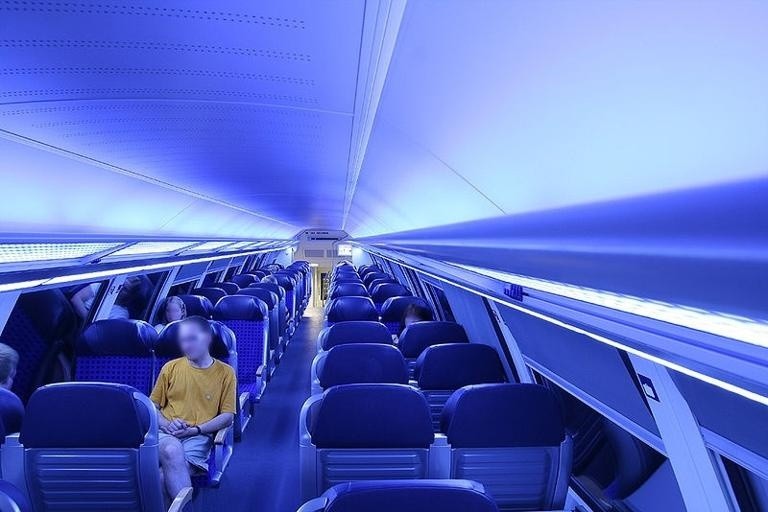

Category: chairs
[157, 297, 206, 323]
[336, 259, 353, 265]
[416, 342, 508, 437]
[363, 267, 384, 279]
[201, 294, 212, 310]
[20, 382, 194, 512]
[153, 316, 235, 489]
[329, 284, 371, 299]
[336, 264, 357, 272]
[315, 322, 396, 346]
[333, 272, 361, 281]
[272, 274, 297, 335]
[233, 273, 256, 290]
[441, 382, 570, 512]
[250, 271, 264, 282]
[298, 261, 314, 315]
[211, 294, 267, 401]
[359, 265, 381, 275]
[247, 283, 286, 364]
[296, 479, 495, 512]
[372, 283, 414, 314]
[369, 279, 401, 293]
[192, 288, 225, 304]
[74, 317, 157, 398]
[378, 297, 436, 342]
[213, 282, 238, 294]
[366, 270, 396, 287]
[236, 287, 279, 378]
[325, 296, 378, 323]
[399, 321, 471, 385]
[218, 321, 259, 441]
[0, 386, 31, 512]
[310, 343, 415, 392]
[330, 279, 366, 285]
[264, 261, 298, 272]
[299, 383, 451, 503]
[250, 282, 298, 348]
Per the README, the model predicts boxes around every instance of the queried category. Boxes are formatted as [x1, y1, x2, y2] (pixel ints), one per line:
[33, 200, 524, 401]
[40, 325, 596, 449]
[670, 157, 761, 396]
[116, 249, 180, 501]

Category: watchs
[196, 426, 201, 435]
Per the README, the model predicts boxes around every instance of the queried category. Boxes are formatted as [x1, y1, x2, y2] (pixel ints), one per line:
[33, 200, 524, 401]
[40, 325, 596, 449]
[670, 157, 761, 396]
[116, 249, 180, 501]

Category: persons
[0, 343, 18, 391]
[164, 296, 187, 325]
[71, 277, 142, 330]
[401, 306, 432, 333]
[149, 316, 236, 512]
